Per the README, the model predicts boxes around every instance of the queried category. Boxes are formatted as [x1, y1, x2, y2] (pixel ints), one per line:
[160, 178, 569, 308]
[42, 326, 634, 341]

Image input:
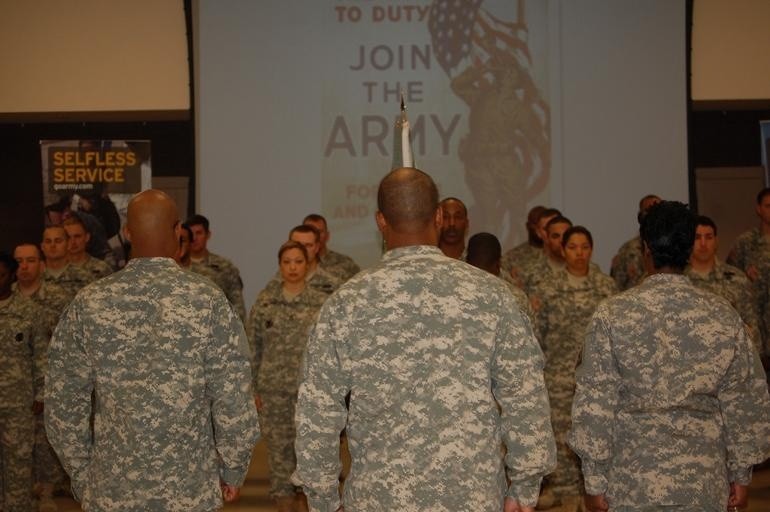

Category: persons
[726, 187, 770, 338]
[520, 216, 602, 508]
[465, 232, 542, 344]
[0, 242, 64, 511]
[288, 167, 558, 512]
[512, 207, 564, 283]
[45, 200, 112, 261]
[10, 240, 73, 502]
[41, 225, 93, 299]
[438, 198, 470, 260]
[610, 194, 663, 293]
[166, 233, 182, 270]
[62, 215, 114, 281]
[501, 205, 548, 280]
[533, 225, 618, 511]
[182, 214, 247, 329]
[246, 241, 329, 512]
[566, 200, 770, 511]
[683, 215, 763, 375]
[44, 189, 261, 512]
[303, 214, 361, 281]
[268, 224, 346, 295]
[173, 223, 231, 302]
